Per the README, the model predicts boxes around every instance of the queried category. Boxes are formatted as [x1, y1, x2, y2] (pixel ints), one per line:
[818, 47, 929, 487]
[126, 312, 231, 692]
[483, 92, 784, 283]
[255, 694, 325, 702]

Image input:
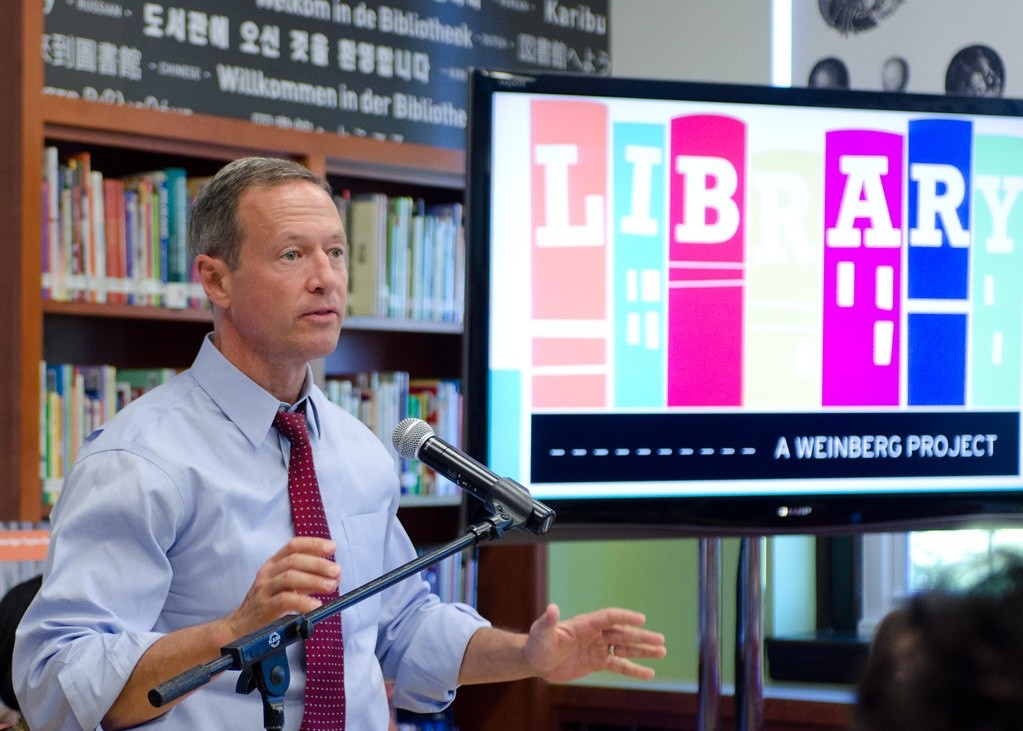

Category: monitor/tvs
[465, 63, 1023, 539]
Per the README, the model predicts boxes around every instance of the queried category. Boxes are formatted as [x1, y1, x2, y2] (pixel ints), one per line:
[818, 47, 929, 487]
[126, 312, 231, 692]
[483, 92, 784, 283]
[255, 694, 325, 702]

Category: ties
[272, 410, 346, 730]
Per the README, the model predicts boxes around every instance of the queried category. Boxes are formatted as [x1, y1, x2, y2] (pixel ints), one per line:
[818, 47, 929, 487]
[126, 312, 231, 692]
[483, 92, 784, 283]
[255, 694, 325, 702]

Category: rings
[609, 645, 616, 656]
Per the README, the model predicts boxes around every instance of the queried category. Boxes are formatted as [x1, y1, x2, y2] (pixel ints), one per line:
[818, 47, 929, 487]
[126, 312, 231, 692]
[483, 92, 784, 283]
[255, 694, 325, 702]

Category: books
[41, 144, 216, 310]
[0, 524, 54, 731]
[408, 541, 477, 614]
[38, 352, 178, 504]
[323, 368, 466, 498]
[329, 185, 466, 323]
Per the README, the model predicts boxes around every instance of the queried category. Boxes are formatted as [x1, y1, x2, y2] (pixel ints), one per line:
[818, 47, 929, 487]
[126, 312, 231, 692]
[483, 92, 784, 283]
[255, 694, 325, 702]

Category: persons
[853, 554, 1023, 731]
[13, 156, 667, 731]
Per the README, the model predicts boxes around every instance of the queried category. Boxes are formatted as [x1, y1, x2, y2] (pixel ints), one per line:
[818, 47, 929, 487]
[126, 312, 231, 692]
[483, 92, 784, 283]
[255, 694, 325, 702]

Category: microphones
[391, 418, 557, 537]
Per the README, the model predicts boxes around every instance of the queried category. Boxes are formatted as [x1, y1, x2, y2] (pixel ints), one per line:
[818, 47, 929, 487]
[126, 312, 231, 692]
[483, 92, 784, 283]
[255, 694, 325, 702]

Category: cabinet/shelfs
[0, 0, 555, 731]
